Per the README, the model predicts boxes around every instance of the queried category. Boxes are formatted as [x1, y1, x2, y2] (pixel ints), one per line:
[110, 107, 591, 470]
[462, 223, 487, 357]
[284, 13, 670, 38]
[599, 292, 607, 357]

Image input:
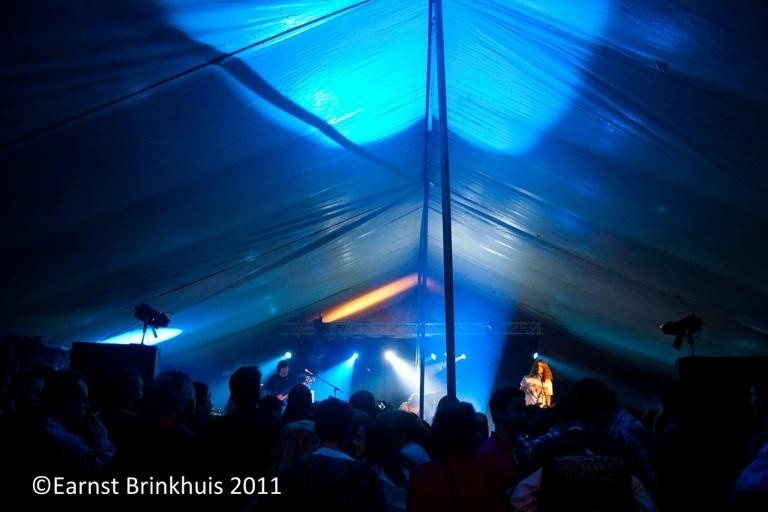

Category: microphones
[304, 368, 315, 376]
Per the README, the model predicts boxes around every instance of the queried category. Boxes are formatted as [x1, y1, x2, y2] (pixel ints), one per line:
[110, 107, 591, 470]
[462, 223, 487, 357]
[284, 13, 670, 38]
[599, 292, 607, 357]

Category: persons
[0, 355, 763, 507]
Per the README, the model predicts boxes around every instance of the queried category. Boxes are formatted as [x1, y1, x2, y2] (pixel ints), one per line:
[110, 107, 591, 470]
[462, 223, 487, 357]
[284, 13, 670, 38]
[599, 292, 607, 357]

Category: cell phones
[376, 399, 388, 414]
